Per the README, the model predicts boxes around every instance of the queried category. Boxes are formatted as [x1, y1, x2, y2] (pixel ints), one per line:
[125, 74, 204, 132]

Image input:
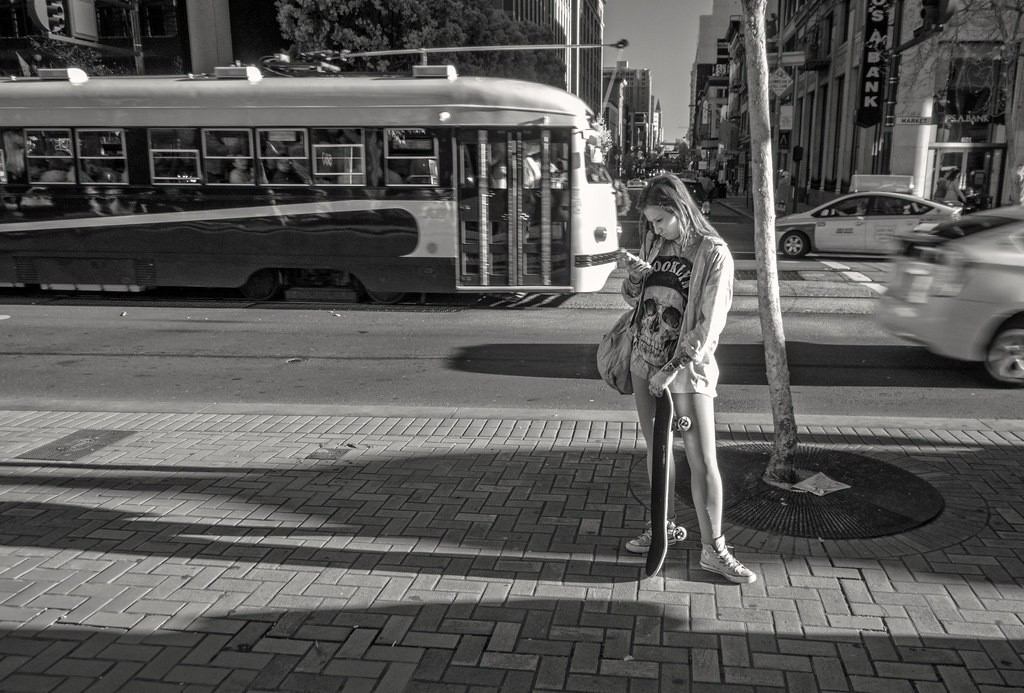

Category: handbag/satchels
[597, 306, 640, 395]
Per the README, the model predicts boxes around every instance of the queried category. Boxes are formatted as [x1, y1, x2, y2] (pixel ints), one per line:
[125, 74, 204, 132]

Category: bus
[0, 66, 621, 306]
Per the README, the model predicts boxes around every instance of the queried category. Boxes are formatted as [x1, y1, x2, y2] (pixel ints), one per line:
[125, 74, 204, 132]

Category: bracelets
[669, 354, 685, 369]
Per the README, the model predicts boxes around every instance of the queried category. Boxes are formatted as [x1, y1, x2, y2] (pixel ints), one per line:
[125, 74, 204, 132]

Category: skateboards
[645, 387, 693, 580]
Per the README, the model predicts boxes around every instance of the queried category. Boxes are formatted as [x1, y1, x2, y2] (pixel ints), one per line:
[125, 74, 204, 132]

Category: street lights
[688, 104, 712, 166]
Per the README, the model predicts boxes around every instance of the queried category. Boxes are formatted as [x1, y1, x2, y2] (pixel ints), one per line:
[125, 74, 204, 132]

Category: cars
[680, 178, 711, 223]
[625, 174, 647, 192]
[648, 171, 655, 177]
[775, 191, 965, 260]
[877, 206, 1024, 390]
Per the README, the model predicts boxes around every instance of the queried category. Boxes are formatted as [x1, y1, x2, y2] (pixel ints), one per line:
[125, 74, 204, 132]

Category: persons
[696, 169, 716, 219]
[934, 168, 982, 216]
[832, 201, 868, 217]
[619, 171, 757, 584]
[3, 133, 561, 243]
[720, 176, 741, 195]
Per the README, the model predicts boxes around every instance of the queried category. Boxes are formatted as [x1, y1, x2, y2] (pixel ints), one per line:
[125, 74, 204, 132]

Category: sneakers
[700, 535, 756, 583]
[626, 520, 676, 552]
